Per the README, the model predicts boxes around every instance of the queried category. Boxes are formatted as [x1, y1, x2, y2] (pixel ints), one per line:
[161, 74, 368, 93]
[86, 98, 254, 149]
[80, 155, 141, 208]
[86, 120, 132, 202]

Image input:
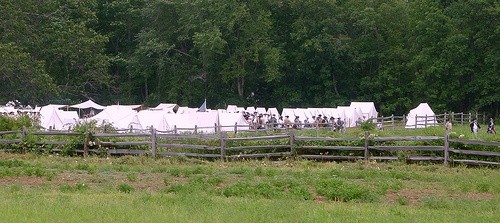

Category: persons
[487, 117, 496, 136]
[470, 119, 482, 139]
[239, 109, 345, 136]
[445, 117, 453, 137]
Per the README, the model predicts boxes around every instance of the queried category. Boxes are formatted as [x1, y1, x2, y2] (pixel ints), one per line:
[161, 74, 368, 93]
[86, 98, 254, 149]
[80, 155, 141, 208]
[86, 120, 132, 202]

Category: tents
[0, 98, 379, 133]
[405, 102, 439, 129]
